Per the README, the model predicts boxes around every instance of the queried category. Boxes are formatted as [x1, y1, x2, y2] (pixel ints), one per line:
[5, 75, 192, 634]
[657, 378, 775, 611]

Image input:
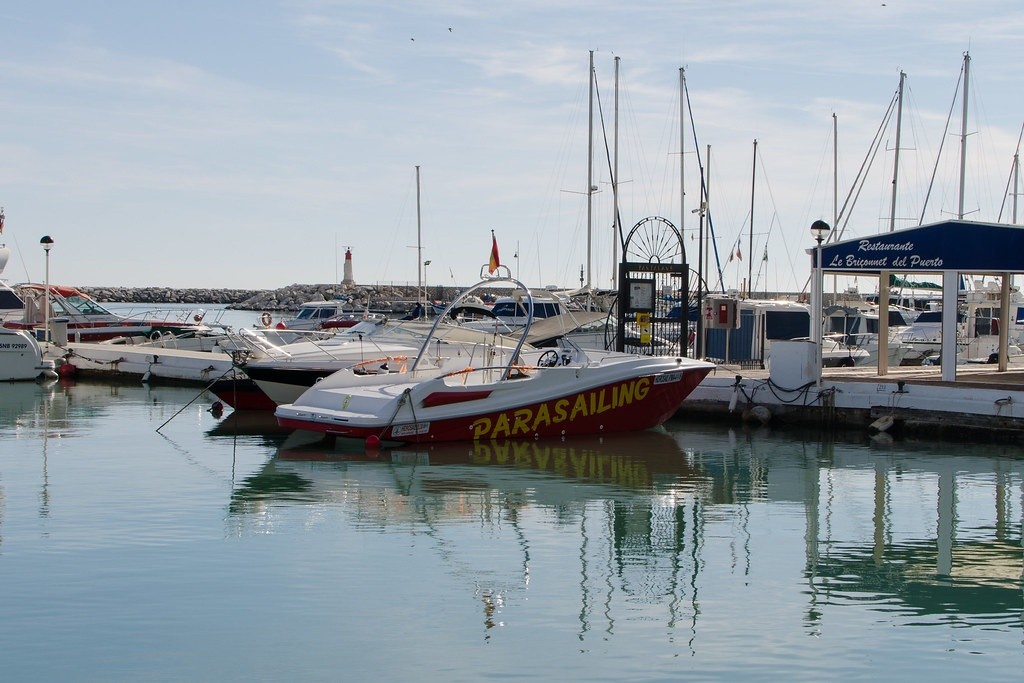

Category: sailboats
[564, 49, 1024, 370]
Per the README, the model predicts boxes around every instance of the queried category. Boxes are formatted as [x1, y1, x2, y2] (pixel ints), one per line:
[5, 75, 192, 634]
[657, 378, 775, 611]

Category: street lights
[808, 220, 830, 386]
[424, 261, 431, 320]
[40, 236, 54, 339]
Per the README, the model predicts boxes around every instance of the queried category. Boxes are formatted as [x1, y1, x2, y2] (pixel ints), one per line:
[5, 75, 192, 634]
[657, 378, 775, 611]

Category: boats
[233, 314, 538, 406]
[1, 166, 695, 382]
[274, 263, 716, 447]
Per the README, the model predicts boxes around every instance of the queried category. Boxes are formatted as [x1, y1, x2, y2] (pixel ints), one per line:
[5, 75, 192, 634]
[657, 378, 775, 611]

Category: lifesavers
[262, 312, 272, 326]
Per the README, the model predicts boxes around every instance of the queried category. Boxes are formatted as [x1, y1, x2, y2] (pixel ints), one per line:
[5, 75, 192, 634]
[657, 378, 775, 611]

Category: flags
[737, 247, 742, 260]
[730, 252, 734, 262]
[489, 237, 499, 274]
